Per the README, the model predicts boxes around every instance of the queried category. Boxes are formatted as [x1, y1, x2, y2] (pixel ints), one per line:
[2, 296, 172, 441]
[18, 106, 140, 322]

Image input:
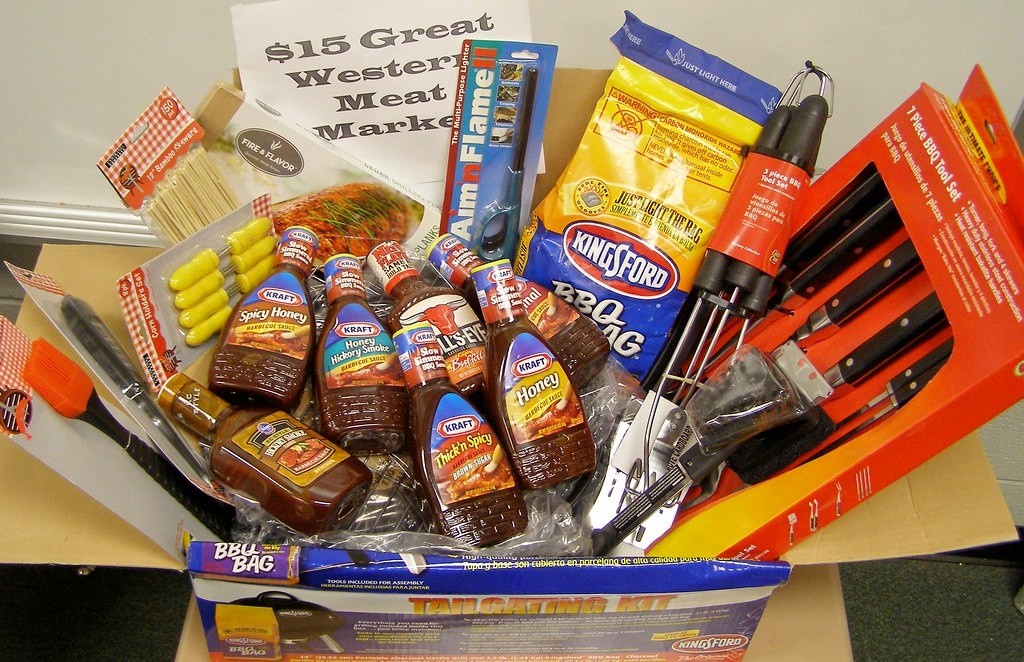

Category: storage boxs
[172, 563, 853, 662]
[186, 538, 791, 662]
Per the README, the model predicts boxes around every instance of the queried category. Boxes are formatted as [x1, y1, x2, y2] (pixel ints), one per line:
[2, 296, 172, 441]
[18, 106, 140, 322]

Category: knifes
[666, 163, 955, 483]
[60, 294, 216, 491]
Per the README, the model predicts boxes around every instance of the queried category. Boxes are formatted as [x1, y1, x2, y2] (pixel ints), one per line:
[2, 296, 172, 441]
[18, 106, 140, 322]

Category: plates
[271, 191, 425, 261]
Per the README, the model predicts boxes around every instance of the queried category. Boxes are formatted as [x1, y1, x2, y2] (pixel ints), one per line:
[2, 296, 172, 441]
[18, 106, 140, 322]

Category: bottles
[158, 226, 610, 548]
